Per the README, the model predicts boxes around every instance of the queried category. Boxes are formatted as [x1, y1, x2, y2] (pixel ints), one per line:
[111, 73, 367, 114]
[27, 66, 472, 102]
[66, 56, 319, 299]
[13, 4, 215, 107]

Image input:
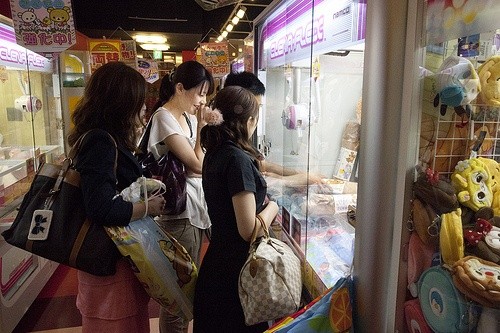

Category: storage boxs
[318, 178, 346, 193]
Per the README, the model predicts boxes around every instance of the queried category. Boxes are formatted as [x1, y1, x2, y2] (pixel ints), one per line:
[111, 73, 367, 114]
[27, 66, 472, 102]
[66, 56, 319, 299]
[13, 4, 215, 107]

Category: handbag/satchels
[2, 128, 117, 276]
[238, 215, 304, 325]
[104, 177, 199, 321]
[133, 111, 187, 217]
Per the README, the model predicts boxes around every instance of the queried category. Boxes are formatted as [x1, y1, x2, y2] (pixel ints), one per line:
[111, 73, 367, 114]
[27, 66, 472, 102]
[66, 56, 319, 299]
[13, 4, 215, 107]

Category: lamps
[216, 5, 247, 41]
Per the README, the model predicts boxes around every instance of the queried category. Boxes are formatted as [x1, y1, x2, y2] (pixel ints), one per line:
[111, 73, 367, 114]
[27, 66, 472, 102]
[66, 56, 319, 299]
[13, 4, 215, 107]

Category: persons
[67, 59, 166, 333]
[141, 61, 213, 333]
[193, 85, 279, 333]
[225, 72, 320, 188]
